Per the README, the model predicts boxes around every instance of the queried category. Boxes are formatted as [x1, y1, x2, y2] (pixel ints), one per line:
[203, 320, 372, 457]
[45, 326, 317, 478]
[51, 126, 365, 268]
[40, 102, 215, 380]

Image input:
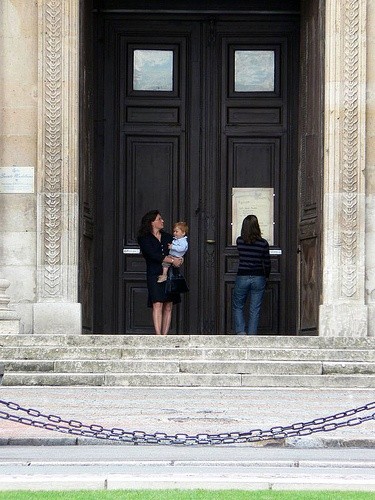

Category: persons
[138, 210, 190, 335]
[233, 215, 271, 335]
[157, 222, 188, 282]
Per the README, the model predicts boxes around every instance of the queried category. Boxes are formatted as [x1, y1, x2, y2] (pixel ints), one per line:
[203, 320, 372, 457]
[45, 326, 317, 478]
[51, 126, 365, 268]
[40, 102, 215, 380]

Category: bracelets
[172, 258, 174, 264]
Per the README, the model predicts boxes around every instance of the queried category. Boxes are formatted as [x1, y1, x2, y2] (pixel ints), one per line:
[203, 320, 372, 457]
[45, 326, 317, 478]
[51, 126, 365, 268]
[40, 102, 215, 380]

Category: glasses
[154, 217, 162, 222]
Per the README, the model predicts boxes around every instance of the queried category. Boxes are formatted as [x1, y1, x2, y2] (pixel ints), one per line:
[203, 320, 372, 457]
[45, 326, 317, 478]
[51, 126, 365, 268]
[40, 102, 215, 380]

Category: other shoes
[157, 275, 167, 283]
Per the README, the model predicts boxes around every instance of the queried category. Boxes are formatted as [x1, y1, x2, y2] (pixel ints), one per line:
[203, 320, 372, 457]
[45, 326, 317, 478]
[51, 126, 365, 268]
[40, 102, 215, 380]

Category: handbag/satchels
[165, 266, 189, 297]
